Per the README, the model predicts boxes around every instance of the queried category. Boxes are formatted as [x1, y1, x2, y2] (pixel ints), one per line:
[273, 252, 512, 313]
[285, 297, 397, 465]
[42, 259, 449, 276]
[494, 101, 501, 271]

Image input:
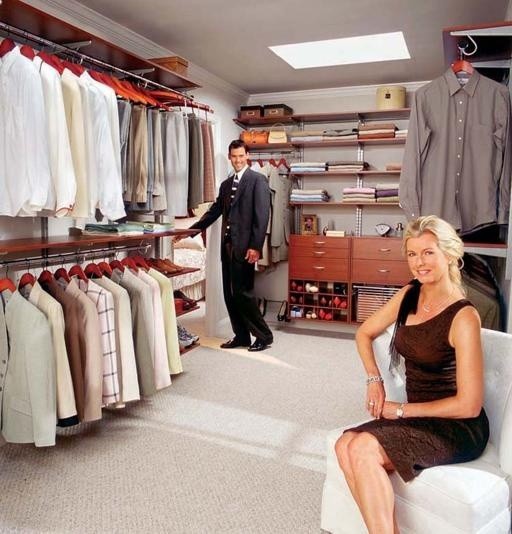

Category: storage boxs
[264, 104, 293, 117]
[239, 106, 263, 118]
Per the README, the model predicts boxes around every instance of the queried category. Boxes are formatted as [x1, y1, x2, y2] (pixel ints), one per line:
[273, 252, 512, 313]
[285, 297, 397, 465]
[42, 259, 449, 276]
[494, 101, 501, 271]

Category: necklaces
[420, 284, 454, 313]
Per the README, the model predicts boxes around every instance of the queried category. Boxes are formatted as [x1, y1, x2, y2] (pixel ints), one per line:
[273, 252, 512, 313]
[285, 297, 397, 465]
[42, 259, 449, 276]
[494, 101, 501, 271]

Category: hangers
[0, 22, 214, 113]
[0, 244, 151, 293]
[450, 47, 475, 75]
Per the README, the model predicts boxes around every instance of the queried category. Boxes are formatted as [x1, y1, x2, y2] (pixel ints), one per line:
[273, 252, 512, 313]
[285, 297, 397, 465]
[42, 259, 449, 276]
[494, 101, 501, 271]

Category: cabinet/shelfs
[234, 109, 412, 325]
[0, 0, 204, 257]
[164, 267, 201, 354]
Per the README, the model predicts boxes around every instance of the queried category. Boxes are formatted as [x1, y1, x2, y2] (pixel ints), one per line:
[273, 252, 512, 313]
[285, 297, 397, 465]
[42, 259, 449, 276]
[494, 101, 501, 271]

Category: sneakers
[178, 326, 199, 348]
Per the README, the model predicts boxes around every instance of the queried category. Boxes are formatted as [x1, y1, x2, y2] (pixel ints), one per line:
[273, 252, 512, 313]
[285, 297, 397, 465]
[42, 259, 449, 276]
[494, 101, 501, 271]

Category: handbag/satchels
[268, 123, 288, 143]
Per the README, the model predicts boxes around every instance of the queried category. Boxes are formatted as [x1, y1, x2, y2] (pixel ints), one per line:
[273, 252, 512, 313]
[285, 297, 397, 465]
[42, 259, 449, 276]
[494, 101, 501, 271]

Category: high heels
[260, 296, 266, 316]
[278, 281, 348, 322]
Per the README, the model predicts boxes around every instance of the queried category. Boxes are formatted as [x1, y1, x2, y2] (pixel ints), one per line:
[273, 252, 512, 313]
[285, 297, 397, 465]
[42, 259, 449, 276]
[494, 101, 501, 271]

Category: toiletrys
[394, 222, 403, 236]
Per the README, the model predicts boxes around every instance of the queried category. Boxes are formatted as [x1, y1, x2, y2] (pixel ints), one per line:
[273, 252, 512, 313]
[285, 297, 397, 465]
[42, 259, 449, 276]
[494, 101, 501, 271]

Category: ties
[225, 173, 239, 236]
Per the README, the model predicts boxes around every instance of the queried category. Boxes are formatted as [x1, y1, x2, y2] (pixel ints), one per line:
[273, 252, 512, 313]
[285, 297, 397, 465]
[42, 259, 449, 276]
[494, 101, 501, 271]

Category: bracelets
[366, 375, 384, 385]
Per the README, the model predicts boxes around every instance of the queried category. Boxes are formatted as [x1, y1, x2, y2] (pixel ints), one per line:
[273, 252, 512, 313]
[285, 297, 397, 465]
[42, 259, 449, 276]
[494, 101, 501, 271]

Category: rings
[369, 401, 374, 406]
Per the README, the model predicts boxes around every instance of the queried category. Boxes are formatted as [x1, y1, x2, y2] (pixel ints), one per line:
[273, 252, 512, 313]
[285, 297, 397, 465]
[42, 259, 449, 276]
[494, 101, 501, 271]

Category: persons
[304, 217, 312, 231]
[335, 213, 490, 533]
[171, 138, 274, 351]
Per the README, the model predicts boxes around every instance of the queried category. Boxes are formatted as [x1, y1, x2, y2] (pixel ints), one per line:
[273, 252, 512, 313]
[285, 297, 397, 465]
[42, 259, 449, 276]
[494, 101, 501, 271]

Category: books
[325, 229, 346, 234]
[325, 233, 345, 236]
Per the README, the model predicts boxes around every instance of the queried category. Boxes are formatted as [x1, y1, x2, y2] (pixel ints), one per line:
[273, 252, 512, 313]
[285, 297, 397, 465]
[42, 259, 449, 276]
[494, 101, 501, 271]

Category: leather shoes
[220, 337, 251, 347]
[174, 291, 197, 310]
[148, 258, 185, 274]
[247, 339, 267, 351]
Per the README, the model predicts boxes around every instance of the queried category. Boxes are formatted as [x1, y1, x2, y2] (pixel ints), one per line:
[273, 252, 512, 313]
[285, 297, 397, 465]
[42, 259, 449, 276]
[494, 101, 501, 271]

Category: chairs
[321, 328, 512, 534]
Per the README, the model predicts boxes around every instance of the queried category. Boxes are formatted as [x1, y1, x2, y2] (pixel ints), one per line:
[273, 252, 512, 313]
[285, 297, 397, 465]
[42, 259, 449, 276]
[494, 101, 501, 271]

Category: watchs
[396, 401, 407, 419]
